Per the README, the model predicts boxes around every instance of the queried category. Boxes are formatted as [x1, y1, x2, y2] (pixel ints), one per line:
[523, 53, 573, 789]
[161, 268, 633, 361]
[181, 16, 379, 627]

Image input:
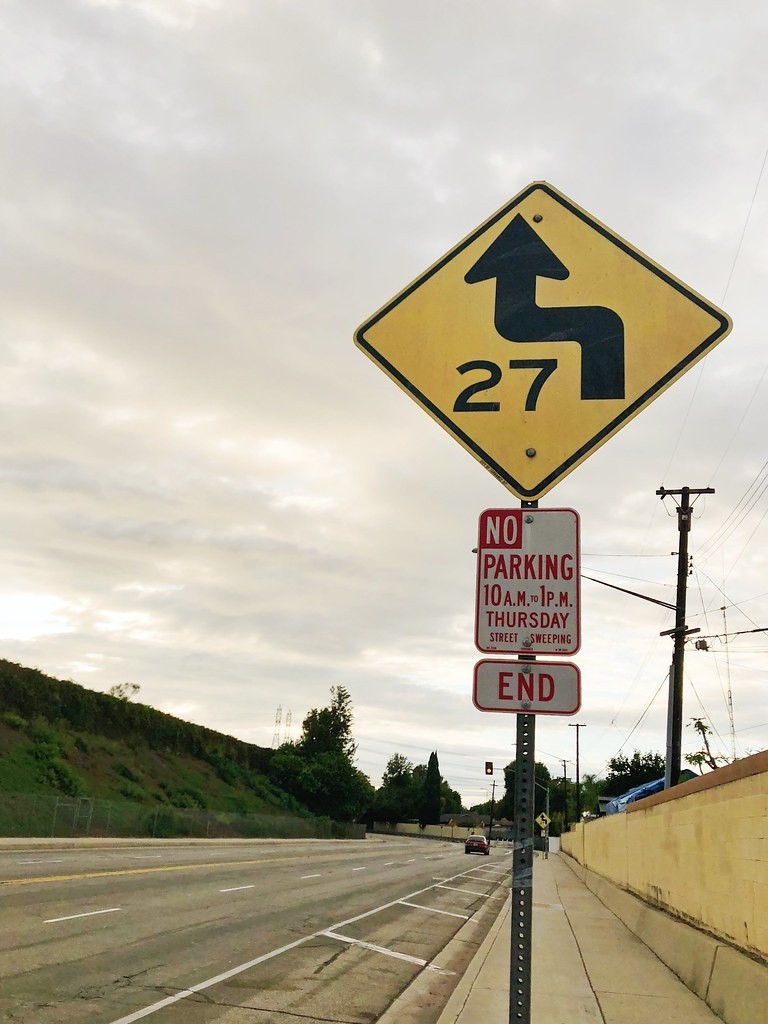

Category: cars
[464, 835, 490, 855]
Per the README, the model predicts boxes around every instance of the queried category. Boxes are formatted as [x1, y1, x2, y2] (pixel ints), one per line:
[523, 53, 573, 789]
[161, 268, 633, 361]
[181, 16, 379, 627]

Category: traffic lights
[485, 761, 494, 776]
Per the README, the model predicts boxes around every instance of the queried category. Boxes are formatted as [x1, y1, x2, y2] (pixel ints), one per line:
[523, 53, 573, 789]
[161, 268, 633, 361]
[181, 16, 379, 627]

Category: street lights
[510, 743, 568, 832]
[569, 723, 587, 823]
